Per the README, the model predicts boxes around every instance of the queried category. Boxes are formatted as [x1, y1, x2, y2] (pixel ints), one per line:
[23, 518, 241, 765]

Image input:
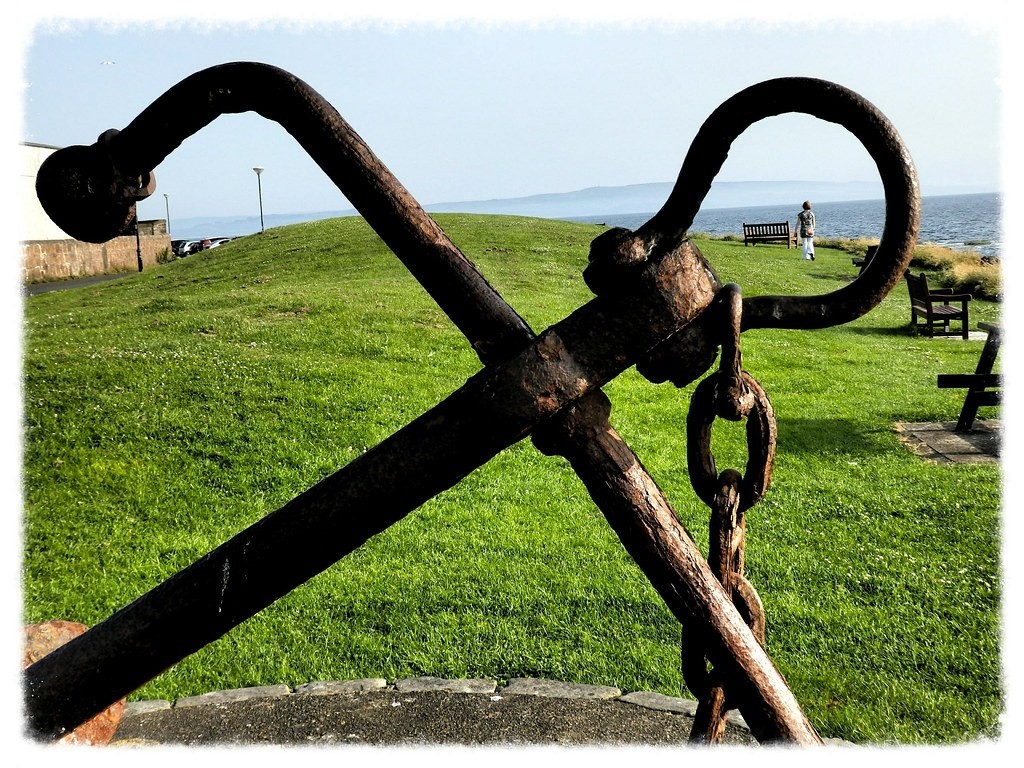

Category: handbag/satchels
[806, 228, 814, 237]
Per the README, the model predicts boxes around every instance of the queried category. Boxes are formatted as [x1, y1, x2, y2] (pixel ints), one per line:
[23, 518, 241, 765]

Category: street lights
[252, 167, 265, 231]
[163, 193, 170, 234]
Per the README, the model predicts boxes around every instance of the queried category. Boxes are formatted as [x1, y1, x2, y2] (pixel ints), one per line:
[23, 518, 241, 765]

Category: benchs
[903, 268, 973, 339]
[851, 257, 866, 265]
[743, 220, 798, 251]
[937, 374, 1001, 389]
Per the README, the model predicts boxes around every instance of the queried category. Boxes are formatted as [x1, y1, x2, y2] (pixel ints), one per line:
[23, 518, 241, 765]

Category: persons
[794, 201, 815, 260]
[190, 242, 201, 254]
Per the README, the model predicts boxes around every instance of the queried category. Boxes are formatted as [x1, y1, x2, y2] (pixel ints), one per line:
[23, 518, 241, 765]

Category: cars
[171, 236, 242, 257]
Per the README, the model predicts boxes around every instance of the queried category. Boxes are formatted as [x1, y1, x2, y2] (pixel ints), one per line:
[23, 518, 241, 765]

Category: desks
[955, 320, 1006, 432]
[858, 245, 877, 275]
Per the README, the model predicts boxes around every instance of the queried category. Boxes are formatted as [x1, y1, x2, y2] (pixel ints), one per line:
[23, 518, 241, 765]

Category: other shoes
[810, 253, 815, 261]
